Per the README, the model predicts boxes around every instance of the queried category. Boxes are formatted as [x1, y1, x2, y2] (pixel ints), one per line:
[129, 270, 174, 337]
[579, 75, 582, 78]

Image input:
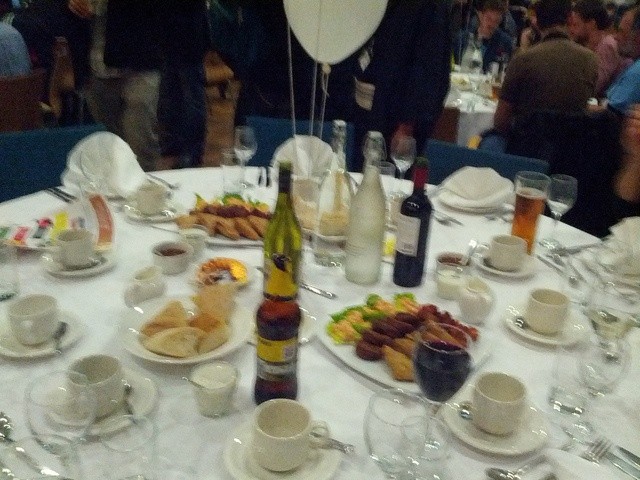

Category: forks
[540, 436, 616, 480]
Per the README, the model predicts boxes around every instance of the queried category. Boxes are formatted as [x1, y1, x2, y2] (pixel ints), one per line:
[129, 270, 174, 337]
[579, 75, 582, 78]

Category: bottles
[261, 160, 305, 304]
[311, 119, 357, 268]
[343, 131, 389, 285]
[254, 255, 302, 405]
[469, 33, 485, 74]
[460, 32, 475, 74]
[392, 157, 434, 287]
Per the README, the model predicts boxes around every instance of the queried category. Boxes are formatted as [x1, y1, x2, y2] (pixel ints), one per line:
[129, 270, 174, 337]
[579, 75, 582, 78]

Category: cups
[510, 171, 551, 255]
[63, 353, 126, 419]
[437, 250, 474, 278]
[187, 360, 240, 418]
[548, 322, 602, 416]
[149, 238, 195, 275]
[124, 265, 166, 306]
[218, 147, 246, 197]
[463, 371, 530, 435]
[0, 432, 84, 476]
[99, 414, 157, 480]
[135, 182, 174, 216]
[7, 293, 61, 346]
[490, 234, 531, 271]
[435, 261, 475, 300]
[0, 239, 23, 304]
[249, 398, 331, 473]
[524, 287, 571, 335]
[174, 223, 213, 264]
[49, 228, 96, 271]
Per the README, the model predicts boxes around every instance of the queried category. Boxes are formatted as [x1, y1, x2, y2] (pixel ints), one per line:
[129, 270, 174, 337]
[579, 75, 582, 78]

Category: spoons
[482, 440, 576, 480]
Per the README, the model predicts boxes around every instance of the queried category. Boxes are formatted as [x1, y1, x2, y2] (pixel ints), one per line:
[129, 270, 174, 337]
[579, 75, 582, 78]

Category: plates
[115, 292, 256, 364]
[430, 213, 438, 232]
[504, 300, 591, 346]
[122, 198, 187, 222]
[315, 292, 499, 405]
[207, 233, 263, 248]
[38, 252, 114, 280]
[440, 383, 552, 455]
[0, 308, 86, 358]
[430, 177, 515, 215]
[245, 302, 319, 346]
[45, 364, 158, 438]
[581, 246, 639, 286]
[477, 250, 539, 277]
[222, 418, 341, 480]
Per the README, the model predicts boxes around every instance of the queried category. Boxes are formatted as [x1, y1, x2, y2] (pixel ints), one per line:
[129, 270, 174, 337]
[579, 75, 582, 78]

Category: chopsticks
[44, 185, 79, 204]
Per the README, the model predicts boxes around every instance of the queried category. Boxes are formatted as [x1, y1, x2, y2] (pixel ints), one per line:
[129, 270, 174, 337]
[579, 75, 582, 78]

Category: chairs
[242, 117, 356, 171]
[0, 122, 109, 204]
[419, 138, 552, 189]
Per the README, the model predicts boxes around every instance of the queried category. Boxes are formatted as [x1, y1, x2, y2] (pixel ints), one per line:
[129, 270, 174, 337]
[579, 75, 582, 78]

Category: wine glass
[24, 369, 98, 480]
[397, 413, 452, 480]
[361, 386, 434, 480]
[410, 323, 475, 460]
[538, 173, 579, 251]
[388, 133, 417, 199]
[588, 274, 640, 364]
[559, 329, 632, 448]
[233, 125, 258, 189]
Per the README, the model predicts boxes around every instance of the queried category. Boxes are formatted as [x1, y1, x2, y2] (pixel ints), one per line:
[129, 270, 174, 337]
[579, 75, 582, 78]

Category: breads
[140, 327, 206, 359]
[142, 300, 188, 341]
[189, 313, 229, 354]
[193, 284, 236, 319]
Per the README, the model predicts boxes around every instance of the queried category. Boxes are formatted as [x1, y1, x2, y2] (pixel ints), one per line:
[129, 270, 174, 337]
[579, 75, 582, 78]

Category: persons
[45, 1, 164, 170]
[456, 0, 512, 77]
[607, 101, 640, 218]
[208, 1, 453, 182]
[591, 4, 640, 127]
[490, 0, 601, 167]
[0, 23, 34, 79]
[572, 0, 634, 97]
[496, 0, 527, 50]
